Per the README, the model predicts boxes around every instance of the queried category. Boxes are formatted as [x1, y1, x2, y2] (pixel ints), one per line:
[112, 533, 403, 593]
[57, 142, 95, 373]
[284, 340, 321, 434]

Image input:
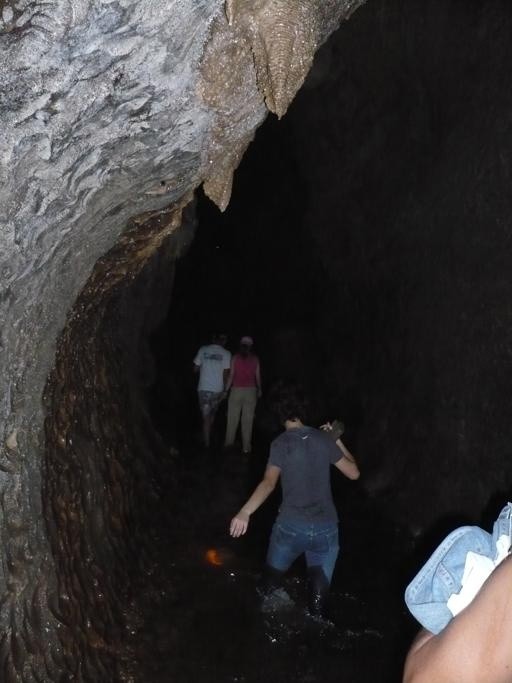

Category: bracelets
[223, 389, 229, 392]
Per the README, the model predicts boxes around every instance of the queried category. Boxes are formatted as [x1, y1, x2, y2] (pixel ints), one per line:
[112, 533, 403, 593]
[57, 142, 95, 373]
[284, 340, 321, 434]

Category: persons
[227, 377, 363, 619]
[221, 334, 264, 454]
[190, 330, 232, 446]
[399, 503, 512, 682]
